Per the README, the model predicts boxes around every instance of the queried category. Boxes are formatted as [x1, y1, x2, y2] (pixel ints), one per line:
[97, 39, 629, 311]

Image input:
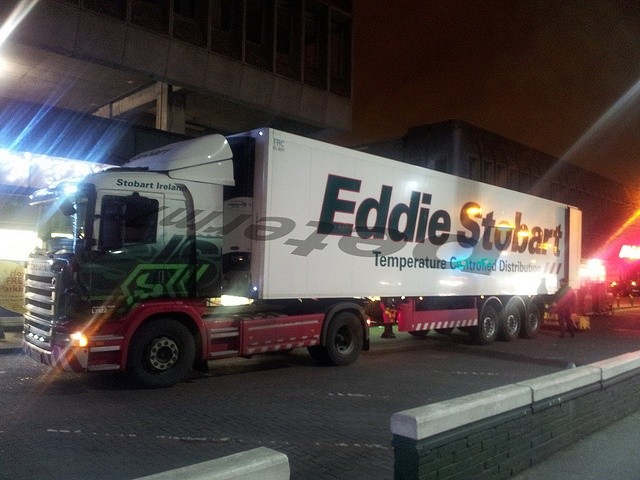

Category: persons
[629, 294, 634, 306]
[554, 279, 576, 338]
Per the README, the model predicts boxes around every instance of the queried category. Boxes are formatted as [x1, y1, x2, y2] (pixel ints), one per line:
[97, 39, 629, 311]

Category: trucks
[22, 127, 583, 390]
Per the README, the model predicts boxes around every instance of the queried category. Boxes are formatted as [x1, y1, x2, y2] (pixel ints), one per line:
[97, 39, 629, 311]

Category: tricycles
[591, 283, 613, 317]
